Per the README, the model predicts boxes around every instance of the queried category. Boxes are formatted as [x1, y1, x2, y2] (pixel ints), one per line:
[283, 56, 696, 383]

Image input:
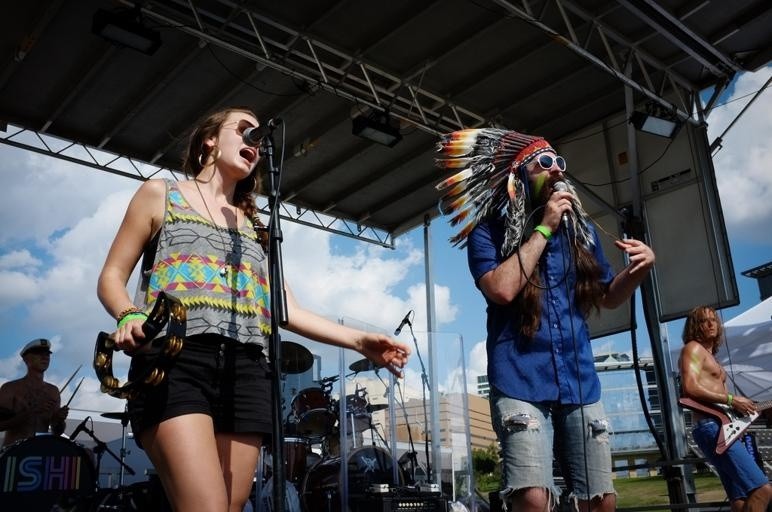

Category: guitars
[677, 397, 772, 455]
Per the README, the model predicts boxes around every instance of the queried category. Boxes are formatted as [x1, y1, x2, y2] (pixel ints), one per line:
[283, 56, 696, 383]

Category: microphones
[242, 120, 279, 145]
[70, 415, 88, 442]
[552, 179, 577, 231]
[394, 308, 415, 336]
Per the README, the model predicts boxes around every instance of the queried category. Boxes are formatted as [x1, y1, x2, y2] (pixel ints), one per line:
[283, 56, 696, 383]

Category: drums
[322, 428, 363, 458]
[301, 446, 406, 511]
[0, 432, 98, 512]
[242, 476, 300, 511]
[283, 437, 310, 484]
[291, 387, 333, 440]
[335, 394, 372, 434]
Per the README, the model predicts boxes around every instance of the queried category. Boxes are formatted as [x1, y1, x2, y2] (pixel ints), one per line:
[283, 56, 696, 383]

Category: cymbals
[349, 358, 385, 371]
[279, 341, 313, 374]
[364, 403, 388, 413]
[100, 413, 130, 419]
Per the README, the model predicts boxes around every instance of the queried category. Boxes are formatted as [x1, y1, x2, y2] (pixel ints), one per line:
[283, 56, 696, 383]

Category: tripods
[100, 427, 139, 511]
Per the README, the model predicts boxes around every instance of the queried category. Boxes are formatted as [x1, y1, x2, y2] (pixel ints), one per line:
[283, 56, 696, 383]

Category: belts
[690, 417, 720, 432]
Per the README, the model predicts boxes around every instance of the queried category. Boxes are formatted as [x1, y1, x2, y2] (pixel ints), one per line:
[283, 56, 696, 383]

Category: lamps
[628, 101, 676, 139]
[352, 113, 404, 150]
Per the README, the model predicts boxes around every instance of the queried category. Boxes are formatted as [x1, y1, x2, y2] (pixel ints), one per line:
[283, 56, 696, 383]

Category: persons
[677, 305, 772, 511]
[432, 127, 656, 512]
[1, 339, 70, 445]
[96, 106, 411, 512]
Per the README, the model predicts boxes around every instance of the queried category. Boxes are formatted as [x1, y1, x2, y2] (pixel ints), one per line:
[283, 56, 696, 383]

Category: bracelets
[727, 394, 733, 405]
[533, 224, 554, 241]
[115, 308, 150, 327]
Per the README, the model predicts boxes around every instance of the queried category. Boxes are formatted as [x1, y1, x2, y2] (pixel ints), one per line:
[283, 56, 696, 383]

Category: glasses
[525, 153, 567, 172]
[222, 119, 269, 153]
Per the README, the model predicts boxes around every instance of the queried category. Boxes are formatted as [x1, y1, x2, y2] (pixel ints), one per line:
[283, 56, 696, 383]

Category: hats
[20, 338, 52, 357]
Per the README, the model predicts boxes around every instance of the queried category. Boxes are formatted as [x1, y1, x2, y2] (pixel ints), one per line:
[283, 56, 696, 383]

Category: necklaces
[193, 176, 239, 277]
